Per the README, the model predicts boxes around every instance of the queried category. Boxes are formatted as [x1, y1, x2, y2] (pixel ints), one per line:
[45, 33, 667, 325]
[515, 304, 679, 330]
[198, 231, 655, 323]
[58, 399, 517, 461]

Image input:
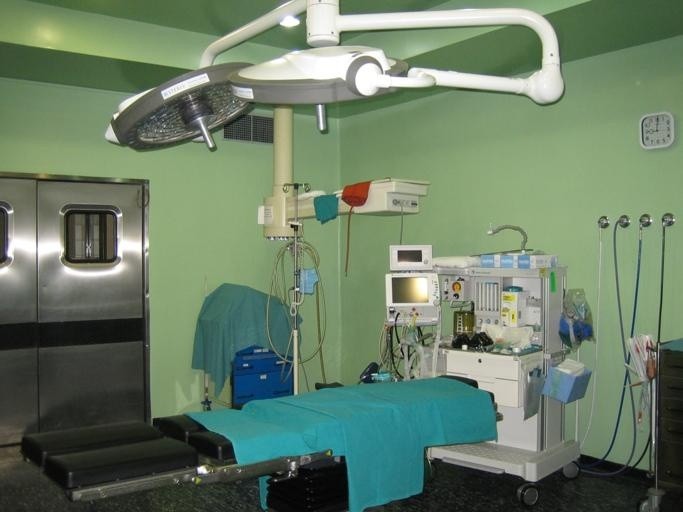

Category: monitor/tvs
[384, 273, 441, 327]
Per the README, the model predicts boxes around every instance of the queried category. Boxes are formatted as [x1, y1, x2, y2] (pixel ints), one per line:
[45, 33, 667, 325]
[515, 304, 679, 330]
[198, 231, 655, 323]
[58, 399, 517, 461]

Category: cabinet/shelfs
[655, 337, 683, 493]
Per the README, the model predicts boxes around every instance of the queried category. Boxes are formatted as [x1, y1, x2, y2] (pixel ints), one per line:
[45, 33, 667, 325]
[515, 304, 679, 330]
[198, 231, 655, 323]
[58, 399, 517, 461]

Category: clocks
[639, 110, 675, 149]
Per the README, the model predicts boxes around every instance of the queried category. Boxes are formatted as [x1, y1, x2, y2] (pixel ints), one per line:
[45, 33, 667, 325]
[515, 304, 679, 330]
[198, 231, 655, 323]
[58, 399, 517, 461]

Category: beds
[20, 375, 502, 512]
